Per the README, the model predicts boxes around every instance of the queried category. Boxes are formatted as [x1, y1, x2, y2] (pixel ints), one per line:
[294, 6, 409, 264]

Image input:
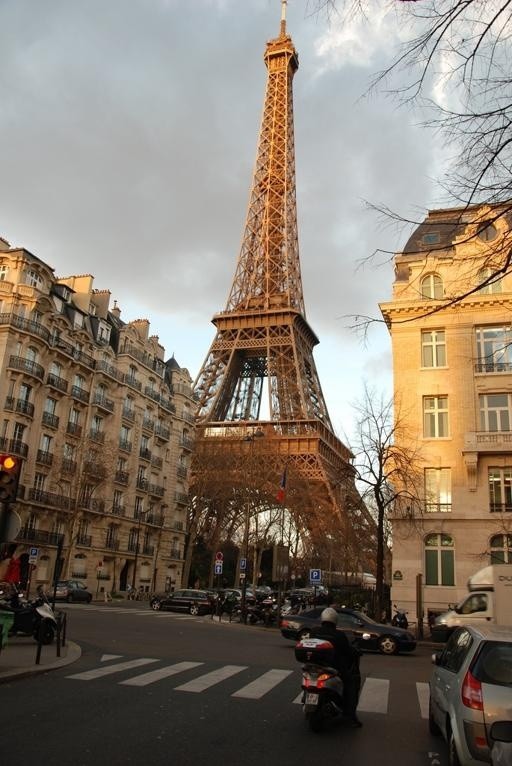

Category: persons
[310, 608, 364, 727]
[128, 583, 144, 601]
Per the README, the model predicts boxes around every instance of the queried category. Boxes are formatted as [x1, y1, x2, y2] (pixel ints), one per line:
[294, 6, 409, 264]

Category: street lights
[134, 502, 169, 591]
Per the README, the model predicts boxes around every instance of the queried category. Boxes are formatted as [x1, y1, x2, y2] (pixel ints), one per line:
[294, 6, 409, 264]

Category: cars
[46, 581, 94, 604]
[488, 720, 512, 746]
[150, 583, 327, 617]
[284, 607, 416, 657]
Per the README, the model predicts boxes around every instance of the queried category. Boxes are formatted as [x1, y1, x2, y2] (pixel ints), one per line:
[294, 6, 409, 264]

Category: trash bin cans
[0, 610, 15, 650]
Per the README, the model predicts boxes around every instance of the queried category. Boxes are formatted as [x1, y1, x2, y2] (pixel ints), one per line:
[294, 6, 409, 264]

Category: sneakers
[343, 715, 363, 729]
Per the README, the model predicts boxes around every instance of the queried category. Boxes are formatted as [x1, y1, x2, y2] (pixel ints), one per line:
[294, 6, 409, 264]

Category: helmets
[320, 607, 338, 624]
[264, 587, 272, 596]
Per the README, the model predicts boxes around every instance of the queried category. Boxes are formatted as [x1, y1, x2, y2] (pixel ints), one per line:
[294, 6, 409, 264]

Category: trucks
[430, 562, 511, 645]
[321, 570, 377, 593]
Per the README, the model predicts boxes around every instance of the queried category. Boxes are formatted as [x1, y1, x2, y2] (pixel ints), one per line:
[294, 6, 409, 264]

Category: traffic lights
[0, 453, 21, 503]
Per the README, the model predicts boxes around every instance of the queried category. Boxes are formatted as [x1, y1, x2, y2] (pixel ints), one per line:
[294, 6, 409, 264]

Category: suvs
[428, 624, 512, 766]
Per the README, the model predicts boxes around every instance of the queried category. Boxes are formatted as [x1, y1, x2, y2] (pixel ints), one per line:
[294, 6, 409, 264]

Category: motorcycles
[0, 581, 57, 648]
[292, 638, 362, 731]
[233, 597, 409, 632]
